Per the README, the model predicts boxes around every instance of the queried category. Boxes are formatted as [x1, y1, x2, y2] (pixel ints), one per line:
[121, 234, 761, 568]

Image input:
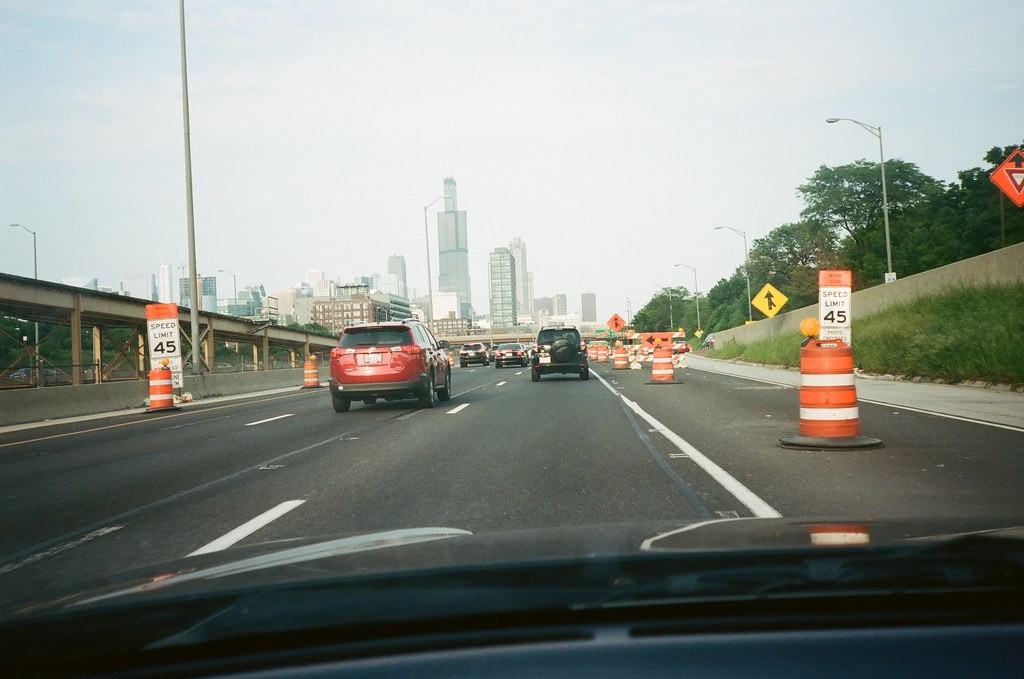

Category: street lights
[376, 306, 387, 322]
[423, 196, 453, 318]
[9, 223, 40, 387]
[675, 264, 701, 329]
[715, 227, 753, 321]
[826, 117, 892, 273]
[218, 269, 239, 362]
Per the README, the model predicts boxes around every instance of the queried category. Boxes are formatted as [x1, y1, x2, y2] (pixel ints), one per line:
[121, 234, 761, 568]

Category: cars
[489, 343, 531, 369]
[671, 341, 690, 354]
[459, 342, 490, 367]
[701, 333, 715, 349]
[9, 368, 63, 384]
[82, 363, 118, 380]
[215, 362, 236, 371]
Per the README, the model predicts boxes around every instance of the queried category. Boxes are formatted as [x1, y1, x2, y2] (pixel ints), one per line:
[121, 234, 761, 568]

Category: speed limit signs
[818, 286, 853, 346]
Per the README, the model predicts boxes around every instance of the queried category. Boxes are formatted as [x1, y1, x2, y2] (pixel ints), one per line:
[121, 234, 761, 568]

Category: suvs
[531, 325, 589, 382]
[328, 318, 452, 413]
